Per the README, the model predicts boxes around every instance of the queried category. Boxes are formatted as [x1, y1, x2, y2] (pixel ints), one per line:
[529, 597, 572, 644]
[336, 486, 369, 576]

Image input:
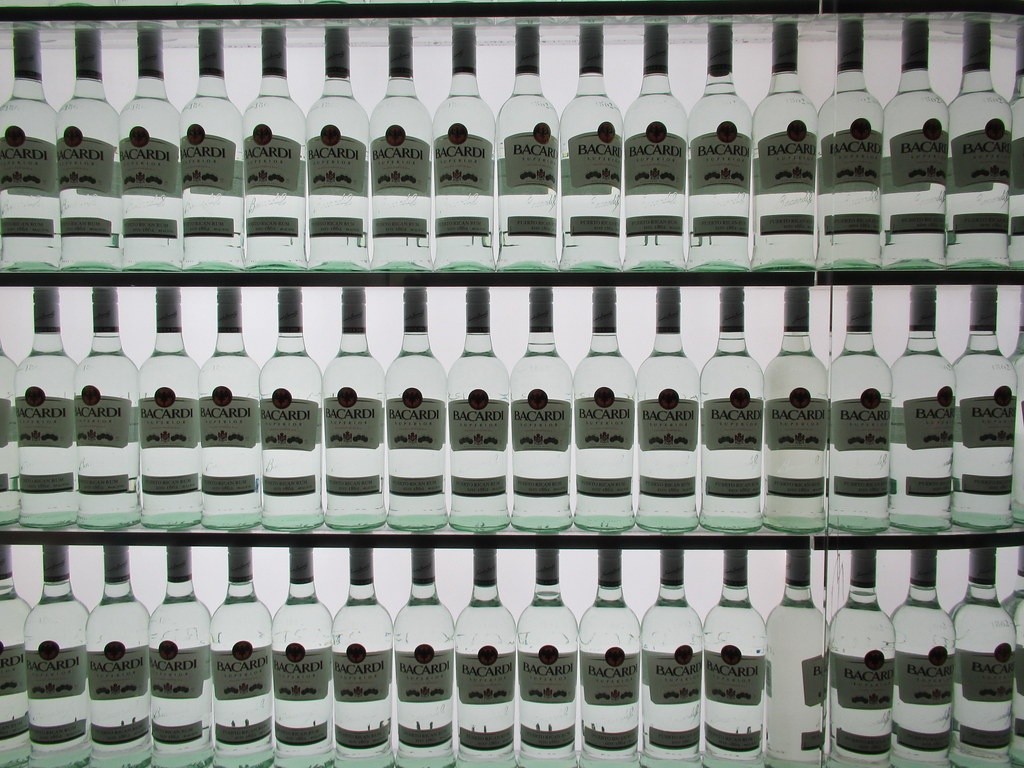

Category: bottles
[198, 287, 263, 531]
[819, 20, 883, 270]
[1, 544, 1024, 768]
[368, 27, 433, 271]
[882, 20, 947, 269]
[1, 20, 62, 271]
[947, 18, 1024, 270]
[308, 28, 373, 274]
[687, 23, 754, 272]
[433, 26, 497, 272]
[0, 286, 79, 529]
[246, 29, 308, 272]
[258, 287, 326, 533]
[623, 26, 687, 272]
[119, 28, 186, 271]
[386, 287, 449, 532]
[75, 287, 142, 530]
[497, 27, 560, 271]
[179, 28, 244, 271]
[324, 287, 386, 530]
[754, 21, 818, 272]
[560, 26, 624, 272]
[58, 28, 125, 272]
[138, 288, 202, 530]
[445, 286, 1023, 534]
